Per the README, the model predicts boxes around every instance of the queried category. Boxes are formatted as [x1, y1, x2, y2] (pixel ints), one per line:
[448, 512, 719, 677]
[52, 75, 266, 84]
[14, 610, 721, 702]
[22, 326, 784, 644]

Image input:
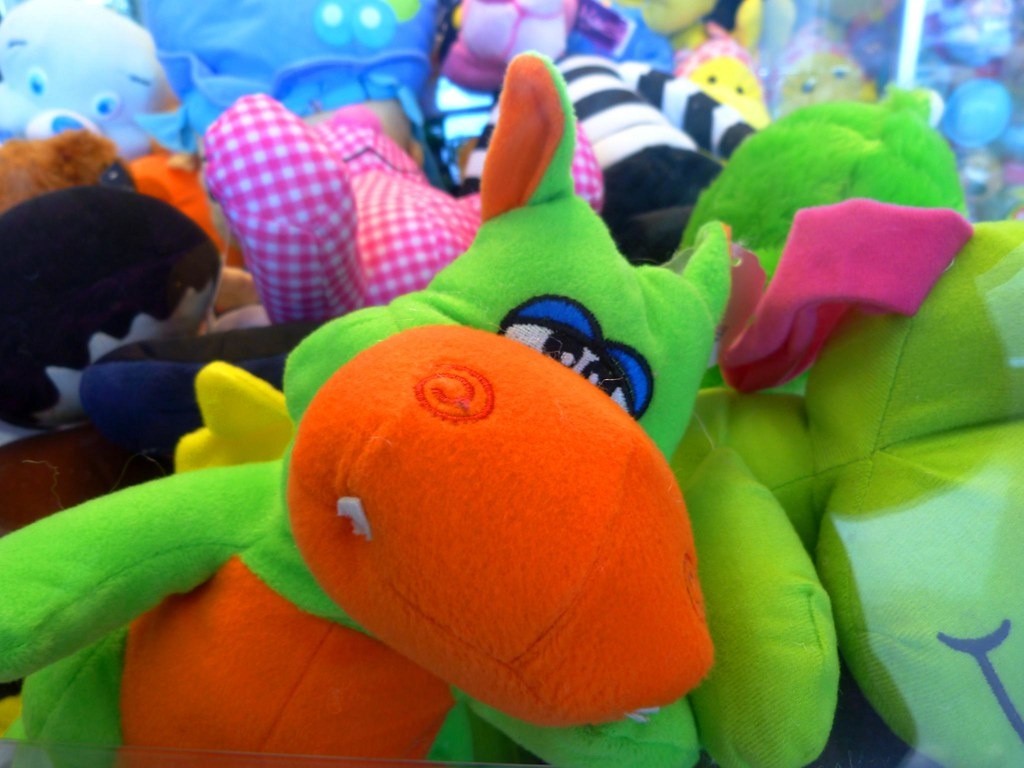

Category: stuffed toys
[0, 51, 731, 768]
[0, 0, 1023, 768]
